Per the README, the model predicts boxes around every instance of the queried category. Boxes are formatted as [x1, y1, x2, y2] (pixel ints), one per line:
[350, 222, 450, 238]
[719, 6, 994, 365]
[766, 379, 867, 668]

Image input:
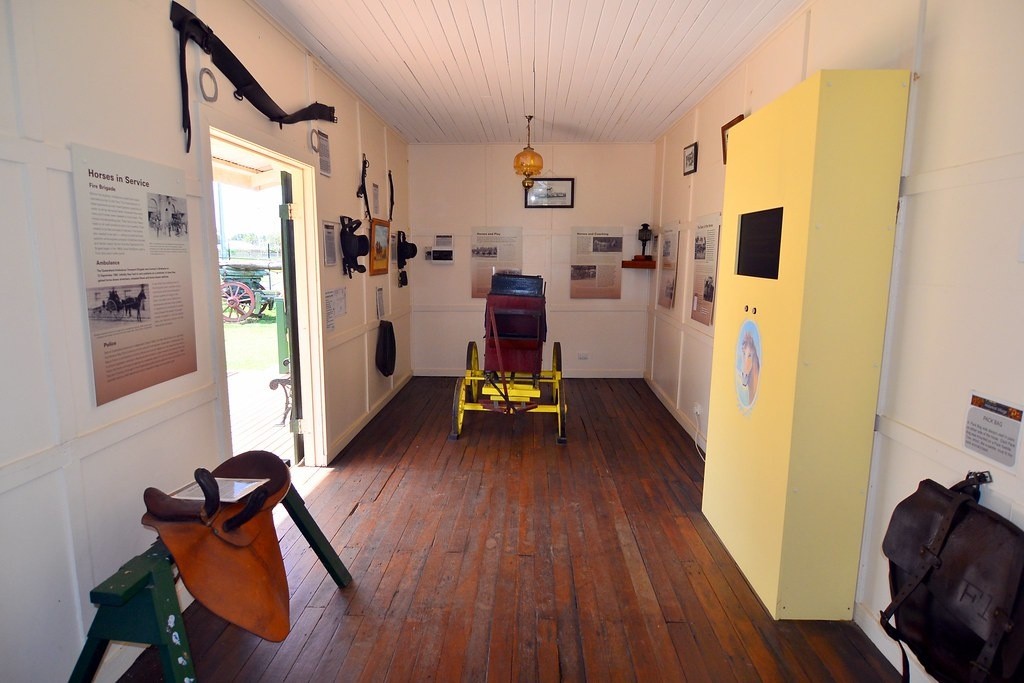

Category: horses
[124, 289, 146, 323]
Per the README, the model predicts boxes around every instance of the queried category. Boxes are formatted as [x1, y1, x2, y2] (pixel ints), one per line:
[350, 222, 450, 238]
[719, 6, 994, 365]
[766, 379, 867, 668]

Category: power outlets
[692, 402, 703, 419]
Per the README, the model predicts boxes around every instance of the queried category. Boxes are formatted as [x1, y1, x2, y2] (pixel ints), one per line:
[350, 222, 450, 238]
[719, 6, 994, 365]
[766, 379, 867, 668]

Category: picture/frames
[682, 142, 698, 175]
[524, 175, 575, 209]
[720, 113, 744, 165]
[368, 217, 391, 275]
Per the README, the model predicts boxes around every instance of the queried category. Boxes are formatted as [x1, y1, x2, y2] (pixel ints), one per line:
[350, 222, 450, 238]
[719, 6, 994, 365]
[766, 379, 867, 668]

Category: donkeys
[741, 332, 760, 404]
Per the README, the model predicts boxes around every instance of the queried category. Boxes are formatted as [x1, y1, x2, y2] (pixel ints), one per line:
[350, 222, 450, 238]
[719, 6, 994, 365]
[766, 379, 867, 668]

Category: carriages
[102, 291, 147, 321]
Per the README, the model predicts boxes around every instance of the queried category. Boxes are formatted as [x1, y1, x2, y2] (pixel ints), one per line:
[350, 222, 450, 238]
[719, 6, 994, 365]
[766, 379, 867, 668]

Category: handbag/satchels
[878, 478, 1023, 683]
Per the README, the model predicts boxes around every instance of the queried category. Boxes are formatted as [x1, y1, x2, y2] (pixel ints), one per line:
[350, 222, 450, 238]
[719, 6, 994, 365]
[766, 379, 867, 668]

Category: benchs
[67, 458, 353, 683]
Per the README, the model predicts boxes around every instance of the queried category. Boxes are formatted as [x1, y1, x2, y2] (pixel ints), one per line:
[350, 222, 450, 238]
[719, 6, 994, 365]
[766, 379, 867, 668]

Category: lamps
[511, 116, 544, 188]
